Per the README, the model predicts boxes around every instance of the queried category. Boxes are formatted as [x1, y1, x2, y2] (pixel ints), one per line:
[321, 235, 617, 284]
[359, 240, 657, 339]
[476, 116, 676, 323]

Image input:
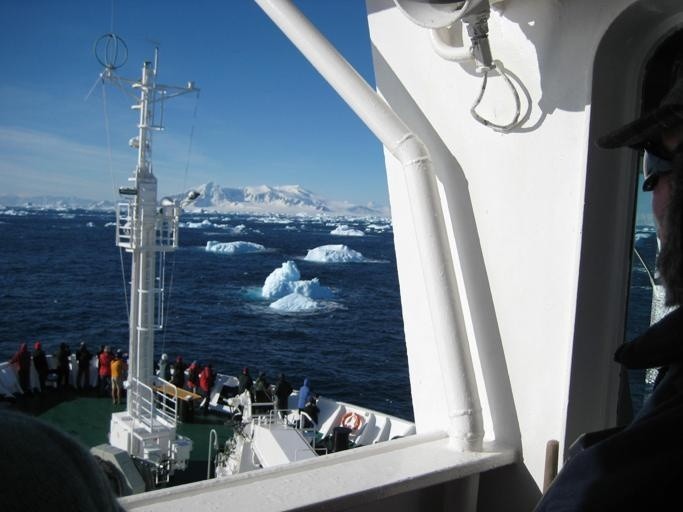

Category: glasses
[640, 134, 683, 192]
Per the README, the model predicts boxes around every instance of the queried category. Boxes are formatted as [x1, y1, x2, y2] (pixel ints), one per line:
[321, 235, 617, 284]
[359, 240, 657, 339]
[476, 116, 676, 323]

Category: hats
[19, 341, 317, 408]
[597, 71, 683, 150]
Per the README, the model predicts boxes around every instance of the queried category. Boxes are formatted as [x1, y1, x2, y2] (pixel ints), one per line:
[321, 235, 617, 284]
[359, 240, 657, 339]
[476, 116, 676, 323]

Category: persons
[234, 367, 321, 429]
[96, 345, 216, 410]
[10, 342, 93, 397]
[531, 66, 681, 512]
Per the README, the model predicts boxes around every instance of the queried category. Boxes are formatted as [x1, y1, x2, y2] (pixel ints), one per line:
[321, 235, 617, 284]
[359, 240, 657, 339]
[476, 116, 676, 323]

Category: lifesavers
[339, 413, 360, 430]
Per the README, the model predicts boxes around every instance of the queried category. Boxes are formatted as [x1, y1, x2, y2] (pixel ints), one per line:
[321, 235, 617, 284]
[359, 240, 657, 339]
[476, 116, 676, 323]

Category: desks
[153, 384, 203, 423]
